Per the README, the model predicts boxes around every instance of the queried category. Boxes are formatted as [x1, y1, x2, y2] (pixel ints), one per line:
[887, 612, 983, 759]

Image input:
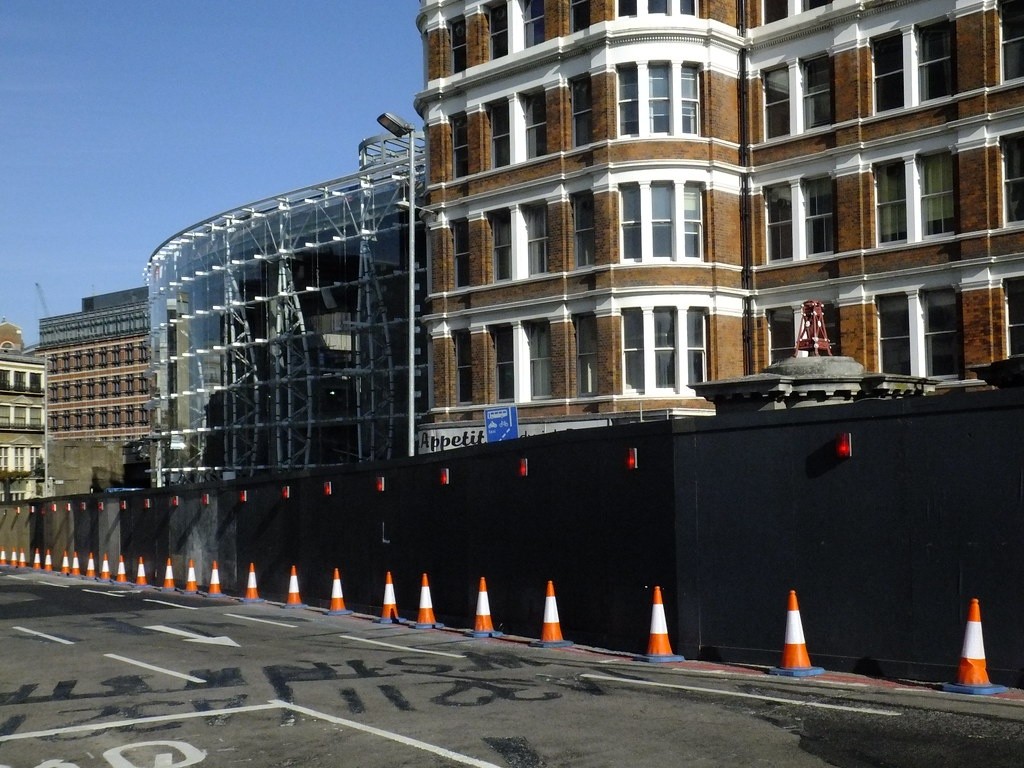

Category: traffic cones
[633, 585, 686, 664]
[28, 547, 44, 572]
[200, 559, 227, 597]
[372, 570, 407, 625]
[109, 554, 133, 585]
[464, 577, 505, 637]
[82, 552, 97, 581]
[408, 573, 444, 629]
[38, 549, 56, 575]
[237, 562, 265, 603]
[280, 565, 307, 610]
[67, 549, 84, 579]
[95, 552, 114, 583]
[178, 558, 204, 594]
[942, 596, 1009, 695]
[7, 546, 19, 570]
[529, 579, 575, 648]
[0, 545, 10, 570]
[130, 556, 152, 588]
[767, 590, 825, 675]
[322, 567, 356, 616]
[16, 548, 32, 572]
[57, 550, 71, 578]
[159, 557, 183, 592]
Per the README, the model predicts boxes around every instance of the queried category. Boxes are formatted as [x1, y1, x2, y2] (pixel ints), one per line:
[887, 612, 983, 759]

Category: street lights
[376, 112, 419, 459]
